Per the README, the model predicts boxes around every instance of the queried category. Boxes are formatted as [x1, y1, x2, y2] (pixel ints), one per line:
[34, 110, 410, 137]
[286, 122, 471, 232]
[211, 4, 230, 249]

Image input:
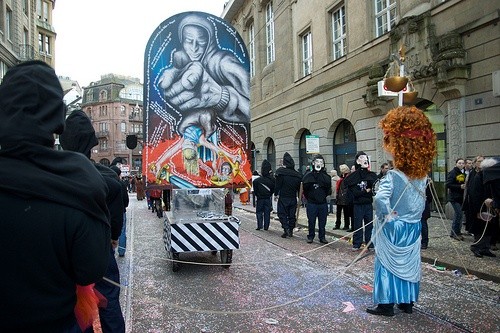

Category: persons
[446, 157, 466, 241]
[239, 170, 261, 208]
[364, 106, 438, 317]
[274, 152, 301, 238]
[59, 108, 171, 333]
[377, 161, 389, 181]
[252, 158, 273, 231]
[462, 155, 500, 257]
[325, 163, 355, 232]
[340, 151, 379, 249]
[422, 174, 434, 250]
[297, 165, 310, 208]
[301, 153, 332, 245]
[272, 172, 278, 214]
[0, 59, 113, 333]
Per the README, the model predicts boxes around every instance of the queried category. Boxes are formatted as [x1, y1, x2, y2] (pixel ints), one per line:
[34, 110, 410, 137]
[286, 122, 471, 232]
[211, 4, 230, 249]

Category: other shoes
[256, 227, 262, 230]
[480, 250, 496, 257]
[281, 232, 289, 237]
[342, 227, 348, 230]
[288, 233, 293, 236]
[264, 228, 267, 230]
[421, 243, 426, 249]
[333, 227, 338, 230]
[471, 248, 482, 257]
[320, 238, 328, 243]
[352, 247, 359, 251]
[346, 229, 352, 232]
[452, 234, 464, 241]
[307, 239, 313, 243]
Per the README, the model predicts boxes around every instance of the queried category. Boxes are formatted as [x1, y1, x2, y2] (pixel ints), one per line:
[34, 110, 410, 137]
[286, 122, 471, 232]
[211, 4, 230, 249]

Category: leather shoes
[367, 306, 394, 316]
[396, 303, 412, 314]
